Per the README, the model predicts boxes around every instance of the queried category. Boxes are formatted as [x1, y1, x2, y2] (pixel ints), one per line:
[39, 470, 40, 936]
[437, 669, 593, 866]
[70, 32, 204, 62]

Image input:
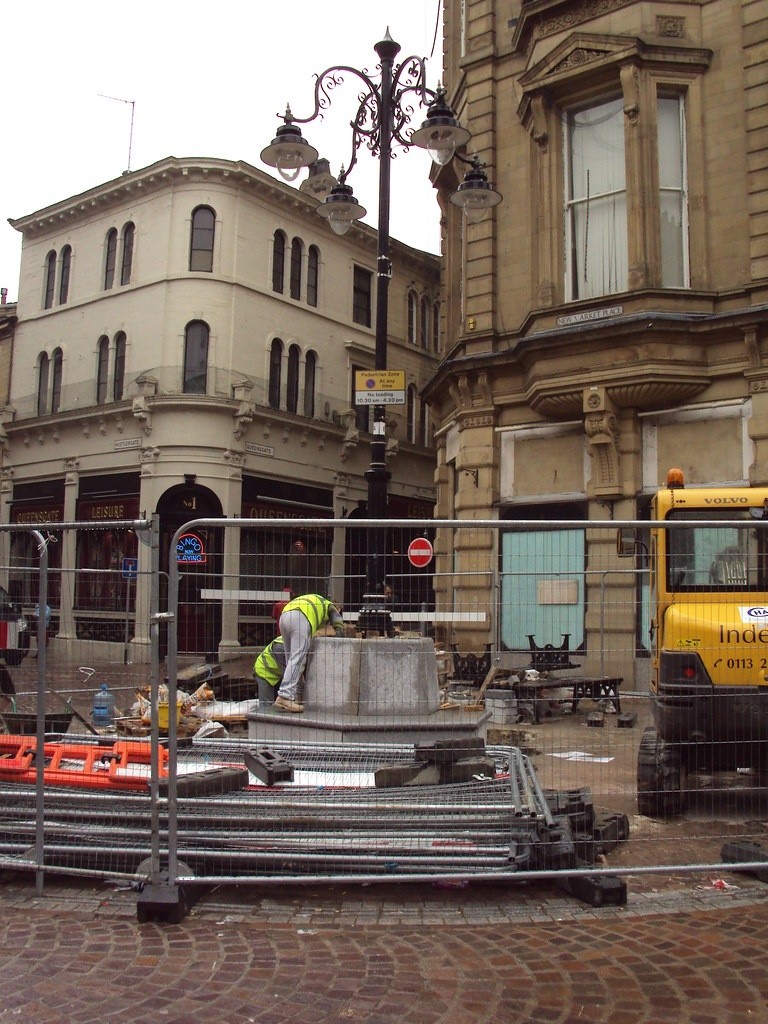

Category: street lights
[258, 26, 506, 631]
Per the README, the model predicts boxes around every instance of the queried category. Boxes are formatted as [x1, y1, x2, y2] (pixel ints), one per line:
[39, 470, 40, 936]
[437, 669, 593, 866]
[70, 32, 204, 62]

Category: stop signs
[408, 537, 433, 568]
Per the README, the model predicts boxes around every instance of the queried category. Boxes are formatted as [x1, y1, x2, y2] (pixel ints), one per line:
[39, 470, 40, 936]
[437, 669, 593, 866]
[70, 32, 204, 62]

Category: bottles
[93, 684, 115, 726]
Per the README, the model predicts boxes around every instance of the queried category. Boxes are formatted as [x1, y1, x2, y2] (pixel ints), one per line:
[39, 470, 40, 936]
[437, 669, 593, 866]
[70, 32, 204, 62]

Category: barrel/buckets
[158, 701, 183, 728]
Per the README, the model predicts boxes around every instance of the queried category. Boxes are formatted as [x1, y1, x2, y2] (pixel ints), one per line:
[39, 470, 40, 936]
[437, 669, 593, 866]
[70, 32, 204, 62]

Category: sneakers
[272, 696, 305, 714]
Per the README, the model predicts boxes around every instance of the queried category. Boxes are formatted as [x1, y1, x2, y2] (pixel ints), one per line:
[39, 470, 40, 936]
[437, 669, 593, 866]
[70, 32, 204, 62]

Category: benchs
[516, 675, 625, 724]
[448, 633, 583, 680]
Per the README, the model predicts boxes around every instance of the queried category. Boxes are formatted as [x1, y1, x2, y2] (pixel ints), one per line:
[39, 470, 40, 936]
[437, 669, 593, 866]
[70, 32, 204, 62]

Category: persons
[255, 636, 286, 708]
[274, 591, 345, 713]
[385, 584, 398, 610]
[32, 601, 51, 660]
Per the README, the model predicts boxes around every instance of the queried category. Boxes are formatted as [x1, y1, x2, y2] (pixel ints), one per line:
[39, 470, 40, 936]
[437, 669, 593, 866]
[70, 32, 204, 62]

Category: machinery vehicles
[615, 466, 767, 821]
